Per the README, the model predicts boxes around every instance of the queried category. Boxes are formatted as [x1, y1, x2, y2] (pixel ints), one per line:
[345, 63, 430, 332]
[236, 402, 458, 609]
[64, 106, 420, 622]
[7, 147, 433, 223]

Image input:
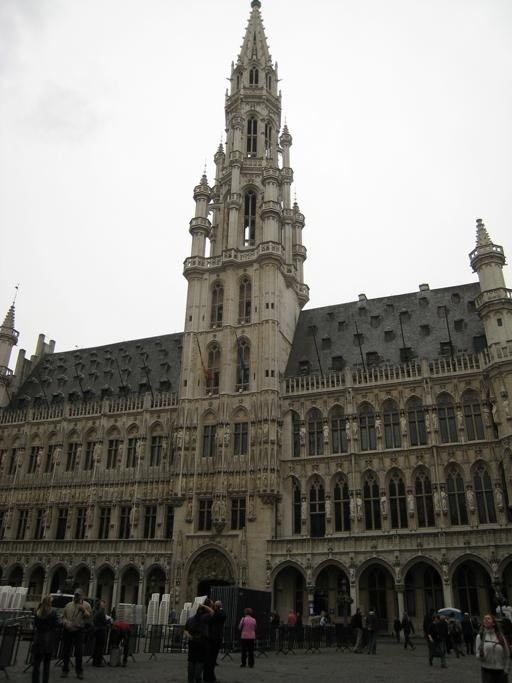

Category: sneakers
[59, 670, 83, 681]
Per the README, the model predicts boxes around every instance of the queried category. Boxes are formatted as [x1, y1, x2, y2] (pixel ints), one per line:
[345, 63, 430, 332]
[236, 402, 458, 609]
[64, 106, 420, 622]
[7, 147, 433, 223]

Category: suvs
[33, 592, 113, 628]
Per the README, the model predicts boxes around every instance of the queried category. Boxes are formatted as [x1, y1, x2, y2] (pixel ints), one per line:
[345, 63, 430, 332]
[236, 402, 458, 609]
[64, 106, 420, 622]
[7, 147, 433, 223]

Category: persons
[351, 608, 379, 653]
[320, 609, 336, 627]
[168, 608, 176, 624]
[31, 590, 131, 682]
[394, 612, 417, 650]
[288, 609, 303, 628]
[184, 600, 226, 683]
[423, 601, 510, 682]
[239, 608, 279, 667]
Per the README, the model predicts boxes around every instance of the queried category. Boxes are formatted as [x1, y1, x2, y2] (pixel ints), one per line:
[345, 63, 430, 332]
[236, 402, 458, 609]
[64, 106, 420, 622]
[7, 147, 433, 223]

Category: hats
[74, 587, 84, 596]
